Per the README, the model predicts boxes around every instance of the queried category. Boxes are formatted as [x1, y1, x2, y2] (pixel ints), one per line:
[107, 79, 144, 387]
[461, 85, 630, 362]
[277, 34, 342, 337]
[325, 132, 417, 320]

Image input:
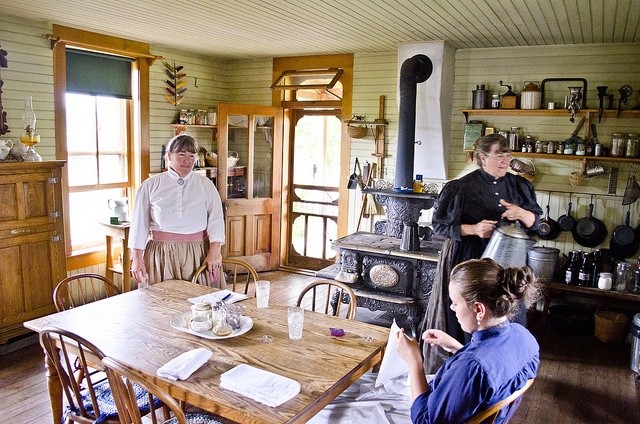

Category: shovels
[347, 158, 358, 189]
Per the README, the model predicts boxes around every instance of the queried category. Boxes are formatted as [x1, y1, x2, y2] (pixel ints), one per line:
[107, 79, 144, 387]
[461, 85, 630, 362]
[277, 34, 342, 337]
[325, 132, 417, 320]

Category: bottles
[565, 248, 600, 288]
[522, 144, 527, 153]
[492, 94, 500, 109]
[527, 145, 532, 153]
[535, 140, 542, 153]
[525, 135, 533, 148]
[22, 95, 36, 132]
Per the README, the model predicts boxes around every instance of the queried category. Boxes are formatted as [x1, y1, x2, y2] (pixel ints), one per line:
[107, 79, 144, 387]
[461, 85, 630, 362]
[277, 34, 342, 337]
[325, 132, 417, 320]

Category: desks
[102, 222, 129, 298]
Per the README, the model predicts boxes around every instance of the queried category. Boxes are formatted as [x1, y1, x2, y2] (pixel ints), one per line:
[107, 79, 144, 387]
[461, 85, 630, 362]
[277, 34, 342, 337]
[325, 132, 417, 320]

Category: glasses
[485, 151, 514, 160]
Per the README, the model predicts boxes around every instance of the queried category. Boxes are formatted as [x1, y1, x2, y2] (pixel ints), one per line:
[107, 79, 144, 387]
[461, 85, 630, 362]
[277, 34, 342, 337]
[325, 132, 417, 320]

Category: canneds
[558, 144, 563, 153]
[202, 110, 207, 124]
[207, 106, 216, 125]
[548, 101, 555, 110]
[179, 108, 189, 125]
[491, 94, 501, 109]
[595, 143, 604, 157]
[196, 109, 204, 124]
[625, 133, 639, 159]
[534, 140, 543, 153]
[609, 132, 625, 157]
[188, 108, 196, 124]
[508, 126, 523, 151]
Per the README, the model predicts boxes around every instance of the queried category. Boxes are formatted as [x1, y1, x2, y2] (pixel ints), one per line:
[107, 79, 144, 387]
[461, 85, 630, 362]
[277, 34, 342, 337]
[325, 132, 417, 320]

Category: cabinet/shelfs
[1, 161, 68, 356]
[461, 108, 640, 161]
[532, 280, 639, 327]
[194, 165, 246, 200]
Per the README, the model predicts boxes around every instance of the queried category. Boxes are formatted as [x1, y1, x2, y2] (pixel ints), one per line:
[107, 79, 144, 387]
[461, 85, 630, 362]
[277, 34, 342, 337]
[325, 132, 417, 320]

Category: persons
[127, 134, 228, 290]
[419, 132, 544, 377]
[304, 256, 541, 424]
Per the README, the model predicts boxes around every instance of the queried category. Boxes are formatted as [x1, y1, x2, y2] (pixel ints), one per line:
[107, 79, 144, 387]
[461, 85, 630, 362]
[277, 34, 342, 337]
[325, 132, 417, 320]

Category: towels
[137, 272, 149, 289]
[220, 362, 301, 409]
[376, 318, 411, 398]
[155, 348, 213, 382]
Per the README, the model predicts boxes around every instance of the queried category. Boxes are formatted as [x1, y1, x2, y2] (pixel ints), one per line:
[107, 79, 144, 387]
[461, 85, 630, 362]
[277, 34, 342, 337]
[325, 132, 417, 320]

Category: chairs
[191, 258, 258, 297]
[39, 327, 128, 423]
[464, 379, 535, 424]
[53, 273, 121, 312]
[296, 279, 356, 321]
[102, 357, 187, 424]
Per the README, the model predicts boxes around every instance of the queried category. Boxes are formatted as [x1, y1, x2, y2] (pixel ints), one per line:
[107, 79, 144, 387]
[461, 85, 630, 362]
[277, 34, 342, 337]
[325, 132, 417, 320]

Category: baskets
[594, 303, 629, 342]
[206, 151, 240, 168]
[346, 116, 368, 138]
[570, 159, 584, 186]
[508, 156, 535, 184]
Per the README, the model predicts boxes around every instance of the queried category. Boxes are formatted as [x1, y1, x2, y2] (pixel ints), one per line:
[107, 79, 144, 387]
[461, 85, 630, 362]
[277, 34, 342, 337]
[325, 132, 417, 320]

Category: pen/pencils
[222, 293, 231, 300]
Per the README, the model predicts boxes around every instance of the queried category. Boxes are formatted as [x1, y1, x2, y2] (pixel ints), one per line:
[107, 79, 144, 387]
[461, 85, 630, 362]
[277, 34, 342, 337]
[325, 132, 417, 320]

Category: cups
[287, 306, 304, 340]
[255, 280, 271, 309]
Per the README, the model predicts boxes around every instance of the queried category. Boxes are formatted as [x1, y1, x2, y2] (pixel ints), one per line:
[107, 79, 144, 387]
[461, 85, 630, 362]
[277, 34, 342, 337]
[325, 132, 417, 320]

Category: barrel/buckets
[527, 246, 560, 283]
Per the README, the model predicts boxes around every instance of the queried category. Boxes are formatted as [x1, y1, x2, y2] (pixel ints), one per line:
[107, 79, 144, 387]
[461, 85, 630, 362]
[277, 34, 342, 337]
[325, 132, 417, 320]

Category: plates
[170, 309, 254, 340]
